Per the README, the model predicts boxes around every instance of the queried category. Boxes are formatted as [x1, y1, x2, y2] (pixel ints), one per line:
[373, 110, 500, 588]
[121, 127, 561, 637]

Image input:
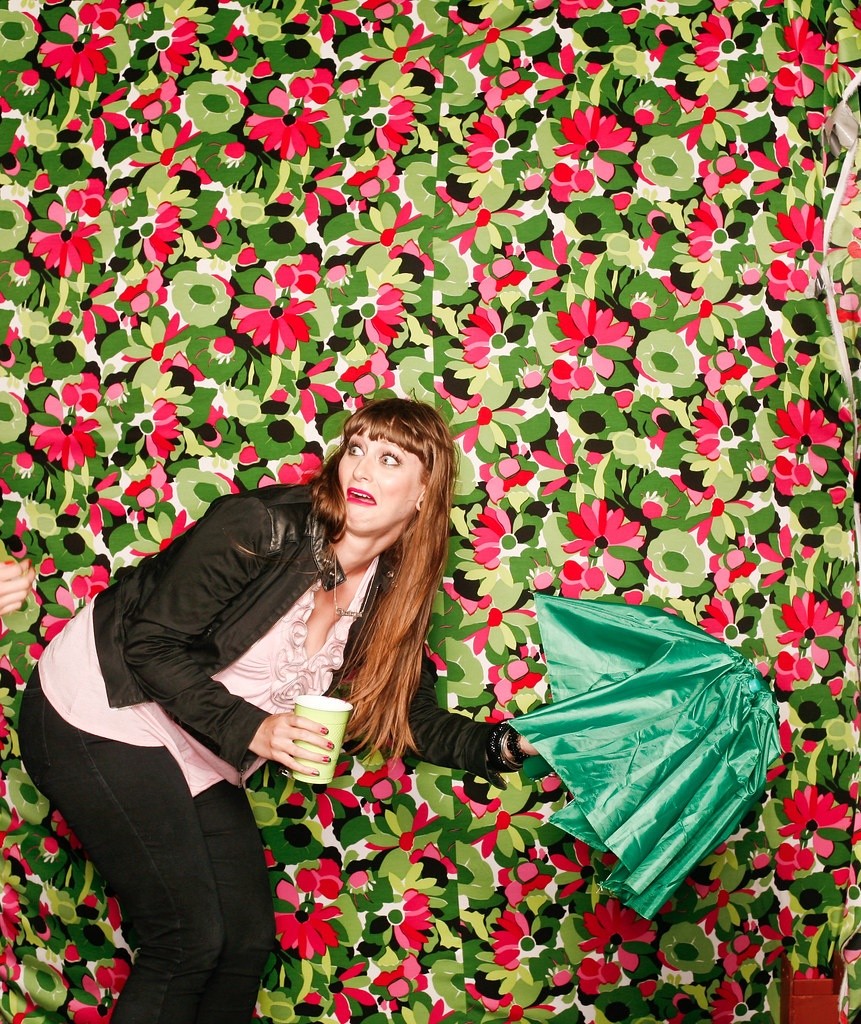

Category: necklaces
[332, 551, 377, 618]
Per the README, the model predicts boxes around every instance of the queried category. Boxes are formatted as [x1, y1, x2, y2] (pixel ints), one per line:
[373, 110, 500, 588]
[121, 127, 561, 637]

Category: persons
[16, 398, 542, 1024]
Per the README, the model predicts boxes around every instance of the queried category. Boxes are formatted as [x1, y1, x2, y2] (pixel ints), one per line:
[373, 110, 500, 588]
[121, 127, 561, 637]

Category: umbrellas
[507, 590, 783, 922]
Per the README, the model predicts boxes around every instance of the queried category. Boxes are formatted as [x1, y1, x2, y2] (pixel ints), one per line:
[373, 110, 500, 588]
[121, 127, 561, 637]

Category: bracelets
[488, 718, 524, 773]
[506, 730, 531, 763]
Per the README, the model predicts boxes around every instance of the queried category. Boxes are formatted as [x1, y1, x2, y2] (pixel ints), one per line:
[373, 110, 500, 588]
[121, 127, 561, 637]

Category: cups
[293, 695, 353, 784]
